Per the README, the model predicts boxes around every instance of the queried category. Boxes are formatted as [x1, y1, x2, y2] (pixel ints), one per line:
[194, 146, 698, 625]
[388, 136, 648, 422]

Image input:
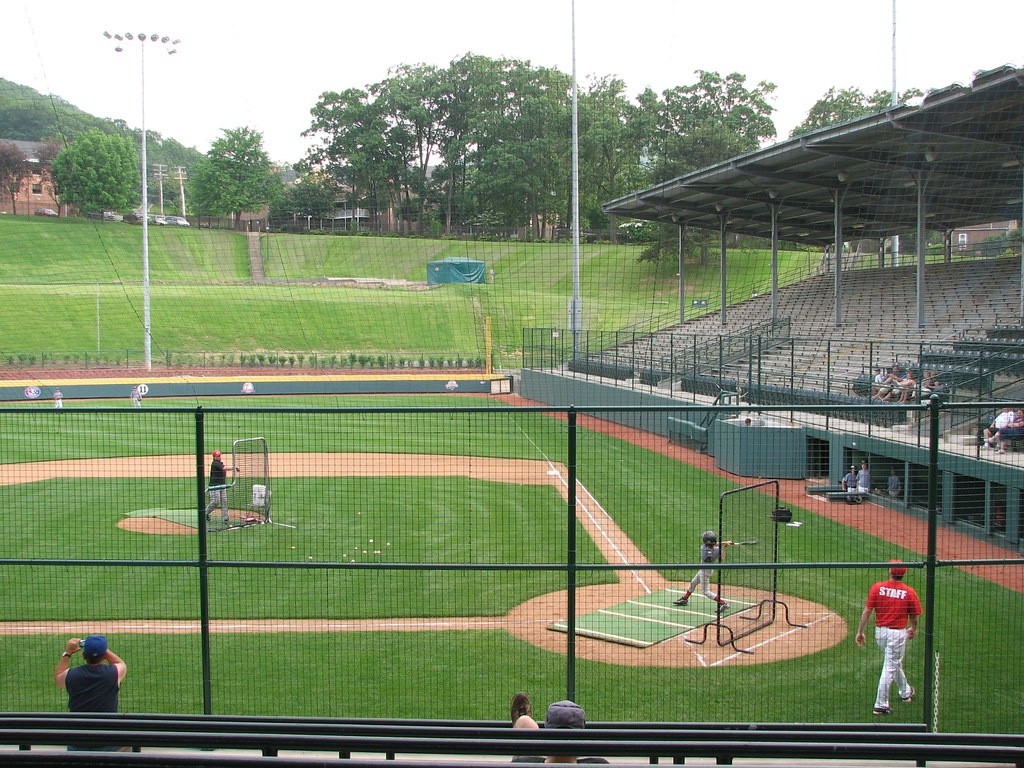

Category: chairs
[566, 325, 1024, 453]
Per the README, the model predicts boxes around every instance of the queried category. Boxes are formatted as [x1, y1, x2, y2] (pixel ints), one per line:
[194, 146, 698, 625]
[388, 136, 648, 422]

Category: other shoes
[205, 514, 210, 521]
[981, 438, 1007, 454]
[225, 521, 233, 528]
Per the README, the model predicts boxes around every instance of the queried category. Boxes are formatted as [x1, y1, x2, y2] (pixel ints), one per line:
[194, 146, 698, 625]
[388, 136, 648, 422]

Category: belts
[847, 486, 856, 488]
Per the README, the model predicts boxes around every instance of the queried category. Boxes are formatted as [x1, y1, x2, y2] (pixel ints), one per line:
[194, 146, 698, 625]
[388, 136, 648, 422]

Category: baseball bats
[724, 541, 759, 548]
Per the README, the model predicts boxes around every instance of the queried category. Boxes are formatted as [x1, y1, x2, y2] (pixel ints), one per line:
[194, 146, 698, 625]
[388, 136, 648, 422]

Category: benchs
[583, 256, 1022, 394]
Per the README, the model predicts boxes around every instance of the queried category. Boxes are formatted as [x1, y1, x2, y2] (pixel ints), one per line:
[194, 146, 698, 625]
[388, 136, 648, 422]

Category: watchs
[62, 652, 71, 657]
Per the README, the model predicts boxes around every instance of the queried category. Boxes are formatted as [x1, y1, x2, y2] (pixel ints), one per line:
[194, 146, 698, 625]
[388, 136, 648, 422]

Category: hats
[889, 559, 907, 576]
[861, 460, 868, 465]
[84, 635, 107, 657]
[891, 465, 898, 470]
[850, 465, 857, 469]
[545, 700, 586, 729]
[212, 450, 221, 457]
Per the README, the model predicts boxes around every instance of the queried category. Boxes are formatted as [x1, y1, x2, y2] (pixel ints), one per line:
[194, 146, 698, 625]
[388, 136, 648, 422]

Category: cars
[34, 208, 58, 216]
[154, 215, 166, 220]
[828, 242, 851, 253]
[168, 218, 190, 227]
[163, 216, 176, 221]
[106, 215, 116, 221]
[152, 218, 169, 227]
[136, 216, 154, 224]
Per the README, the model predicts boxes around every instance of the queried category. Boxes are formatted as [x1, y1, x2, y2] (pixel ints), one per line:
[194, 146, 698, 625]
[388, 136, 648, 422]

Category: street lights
[103, 30, 181, 371]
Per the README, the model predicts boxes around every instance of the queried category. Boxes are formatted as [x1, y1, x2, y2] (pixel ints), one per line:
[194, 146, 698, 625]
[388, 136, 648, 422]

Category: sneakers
[902, 687, 914, 702]
[873, 707, 891, 715]
[511, 692, 531, 726]
[673, 597, 688, 605]
[715, 602, 728, 613]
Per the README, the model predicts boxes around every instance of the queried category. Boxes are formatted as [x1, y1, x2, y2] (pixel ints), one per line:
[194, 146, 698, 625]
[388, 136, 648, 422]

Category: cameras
[79, 640, 85, 647]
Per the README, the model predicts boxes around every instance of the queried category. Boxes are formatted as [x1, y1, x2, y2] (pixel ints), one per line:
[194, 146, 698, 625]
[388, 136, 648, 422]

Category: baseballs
[343, 554, 346, 557]
[354, 547, 358, 549]
[309, 556, 313, 560]
[229, 525, 233, 527]
[374, 550, 377, 553]
[292, 546, 295, 549]
[358, 512, 361, 515]
[369, 539, 373, 542]
[261, 522, 264, 524]
[378, 550, 380, 553]
[351, 560, 355, 562]
[387, 543, 390, 546]
[363, 551, 366, 553]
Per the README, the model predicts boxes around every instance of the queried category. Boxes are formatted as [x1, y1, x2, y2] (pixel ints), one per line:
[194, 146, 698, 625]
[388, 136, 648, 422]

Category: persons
[887, 465, 902, 498]
[871, 365, 940, 403]
[856, 560, 922, 714]
[983, 407, 1024, 453]
[130, 386, 142, 406]
[205, 450, 239, 523]
[842, 458, 870, 494]
[510, 693, 609, 765]
[54, 634, 132, 752]
[673, 531, 732, 611]
[53, 388, 64, 408]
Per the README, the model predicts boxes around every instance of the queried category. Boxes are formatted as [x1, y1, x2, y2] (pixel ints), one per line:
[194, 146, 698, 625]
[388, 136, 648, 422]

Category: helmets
[703, 531, 717, 546]
